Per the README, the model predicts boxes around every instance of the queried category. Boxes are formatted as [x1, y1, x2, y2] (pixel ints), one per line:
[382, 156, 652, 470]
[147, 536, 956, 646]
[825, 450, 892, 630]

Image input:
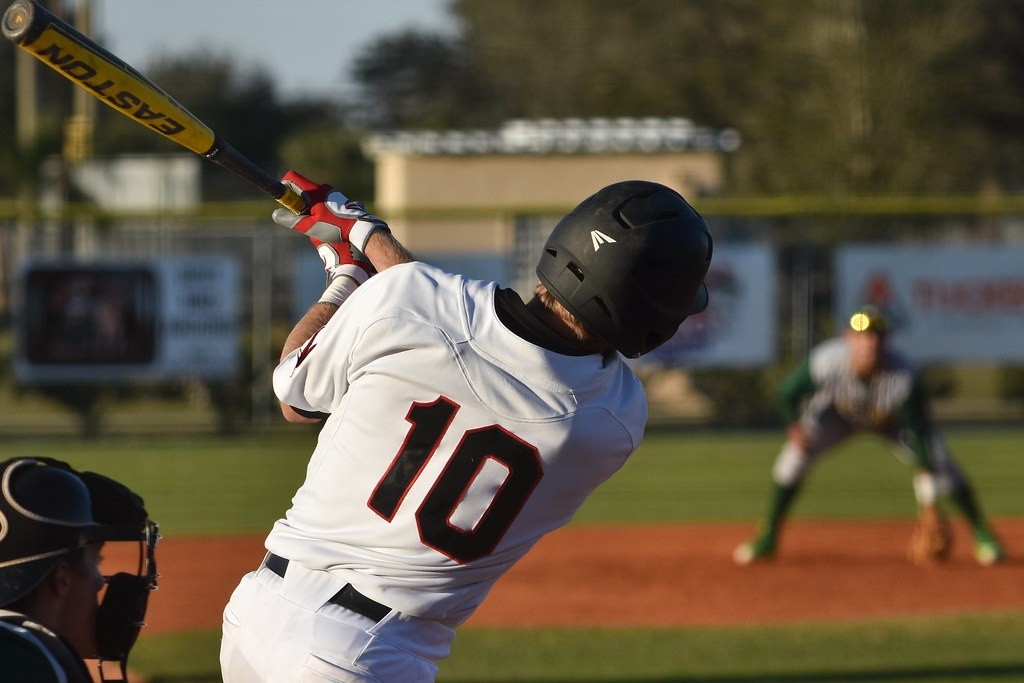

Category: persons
[219, 170, 713, 683]
[0, 455, 160, 683]
[734, 309, 1003, 564]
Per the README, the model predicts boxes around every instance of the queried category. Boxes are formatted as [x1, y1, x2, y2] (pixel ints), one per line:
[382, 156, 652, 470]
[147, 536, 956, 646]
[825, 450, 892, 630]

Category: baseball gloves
[898, 511, 955, 563]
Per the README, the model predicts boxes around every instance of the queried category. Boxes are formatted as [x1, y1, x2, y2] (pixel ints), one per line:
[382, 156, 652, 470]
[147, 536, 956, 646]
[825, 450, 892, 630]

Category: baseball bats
[0, 1, 310, 220]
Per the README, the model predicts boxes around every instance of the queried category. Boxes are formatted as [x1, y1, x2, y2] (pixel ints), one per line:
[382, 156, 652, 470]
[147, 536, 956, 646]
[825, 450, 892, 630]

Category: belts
[264, 551, 393, 623]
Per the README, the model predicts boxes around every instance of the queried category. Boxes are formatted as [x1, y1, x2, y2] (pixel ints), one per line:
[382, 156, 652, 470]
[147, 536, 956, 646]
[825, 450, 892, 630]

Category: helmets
[0, 457, 97, 606]
[535, 180, 713, 360]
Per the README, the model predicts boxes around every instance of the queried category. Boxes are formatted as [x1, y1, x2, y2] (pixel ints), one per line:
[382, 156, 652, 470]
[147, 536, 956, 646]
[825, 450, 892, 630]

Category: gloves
[272, 170, 392, 254]
[310, 237, 376, 306]
